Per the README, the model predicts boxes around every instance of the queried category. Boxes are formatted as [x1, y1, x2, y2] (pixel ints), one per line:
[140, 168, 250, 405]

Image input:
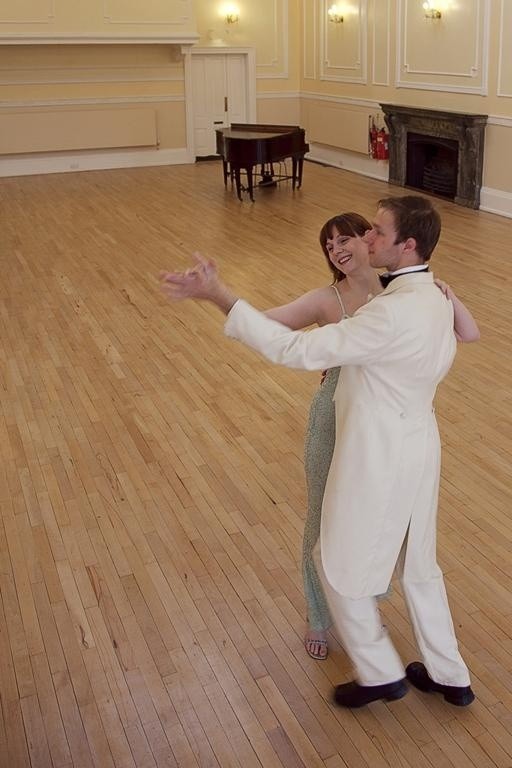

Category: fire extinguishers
[368, 115, 389, 160]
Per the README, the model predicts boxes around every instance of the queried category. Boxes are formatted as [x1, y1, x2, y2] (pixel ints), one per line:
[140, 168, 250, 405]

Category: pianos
[215, 123, 309, 202]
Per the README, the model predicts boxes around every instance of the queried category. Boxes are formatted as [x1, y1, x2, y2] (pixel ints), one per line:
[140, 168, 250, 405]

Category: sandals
[304, 624, 329, 662]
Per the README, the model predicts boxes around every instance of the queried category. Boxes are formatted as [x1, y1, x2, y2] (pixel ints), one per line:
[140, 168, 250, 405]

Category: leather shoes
[404, 661, 475, 707]
[332, 677, 409, 708]
